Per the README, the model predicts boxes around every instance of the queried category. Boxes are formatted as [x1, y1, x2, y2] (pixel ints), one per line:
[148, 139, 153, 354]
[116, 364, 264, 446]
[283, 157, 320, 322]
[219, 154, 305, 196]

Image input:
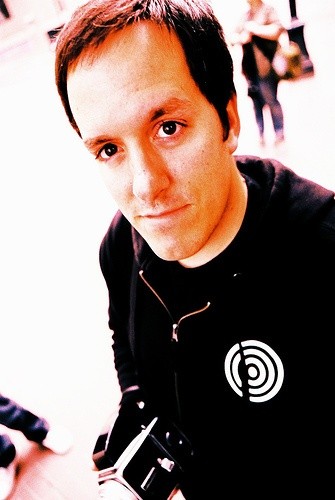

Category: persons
[0, 393, 73, 500]
[55, 0, 335, 500]
[232, 0, 290, 147]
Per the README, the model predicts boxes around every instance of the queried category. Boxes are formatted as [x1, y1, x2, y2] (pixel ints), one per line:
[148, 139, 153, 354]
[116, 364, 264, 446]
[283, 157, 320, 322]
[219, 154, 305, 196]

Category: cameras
[91, 410, 197, 500]
